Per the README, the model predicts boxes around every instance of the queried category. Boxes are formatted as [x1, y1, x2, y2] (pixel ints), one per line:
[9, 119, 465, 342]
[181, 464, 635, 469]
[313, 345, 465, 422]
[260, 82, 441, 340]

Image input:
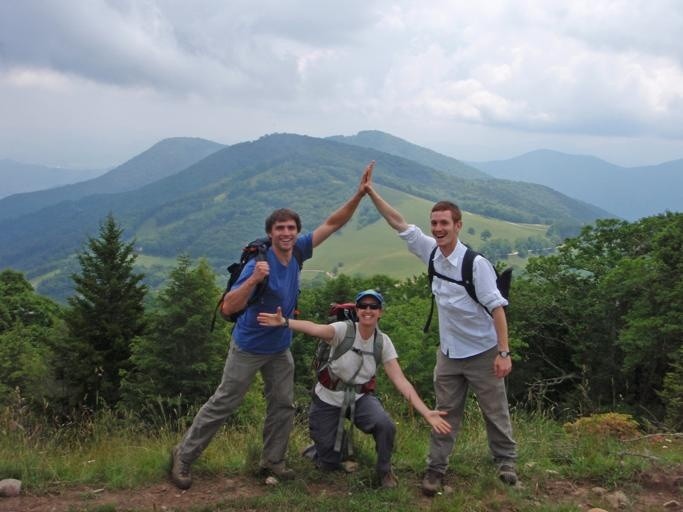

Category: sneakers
[171, 445, 192, 490]
[379, 474, 398, 489]
[259, 460, 296, 481]
[497, 465, 518, 486]
[422, 470, 443, 496]
[302, 443, 317, 460]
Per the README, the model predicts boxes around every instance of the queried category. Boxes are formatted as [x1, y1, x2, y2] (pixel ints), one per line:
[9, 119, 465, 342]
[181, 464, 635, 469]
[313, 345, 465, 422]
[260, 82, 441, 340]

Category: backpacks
[210, 235, 303, 333]
[423, 241, 513, 333]
[313, 303, 383, 394]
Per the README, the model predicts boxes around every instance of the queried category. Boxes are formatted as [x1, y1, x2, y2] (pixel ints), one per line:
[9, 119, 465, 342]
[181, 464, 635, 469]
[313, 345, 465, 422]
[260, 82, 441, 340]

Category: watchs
[498, 350, 511, 358]
[283, 316, 289, 330]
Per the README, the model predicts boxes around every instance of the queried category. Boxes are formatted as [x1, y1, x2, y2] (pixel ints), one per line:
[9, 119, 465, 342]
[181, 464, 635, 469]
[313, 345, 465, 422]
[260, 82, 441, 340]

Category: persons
[172, 164, 368, 491]
[256, 290, 451, 489]
[366, 160, 519, 495]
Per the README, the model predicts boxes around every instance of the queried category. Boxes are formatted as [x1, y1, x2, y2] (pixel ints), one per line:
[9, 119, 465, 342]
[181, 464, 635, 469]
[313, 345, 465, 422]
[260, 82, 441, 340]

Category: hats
[355, 289, 384, 303]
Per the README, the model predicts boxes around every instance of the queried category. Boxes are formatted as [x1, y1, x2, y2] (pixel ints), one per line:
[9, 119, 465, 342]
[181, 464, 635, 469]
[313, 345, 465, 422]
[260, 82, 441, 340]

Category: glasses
[359, 304, 378, 309]
